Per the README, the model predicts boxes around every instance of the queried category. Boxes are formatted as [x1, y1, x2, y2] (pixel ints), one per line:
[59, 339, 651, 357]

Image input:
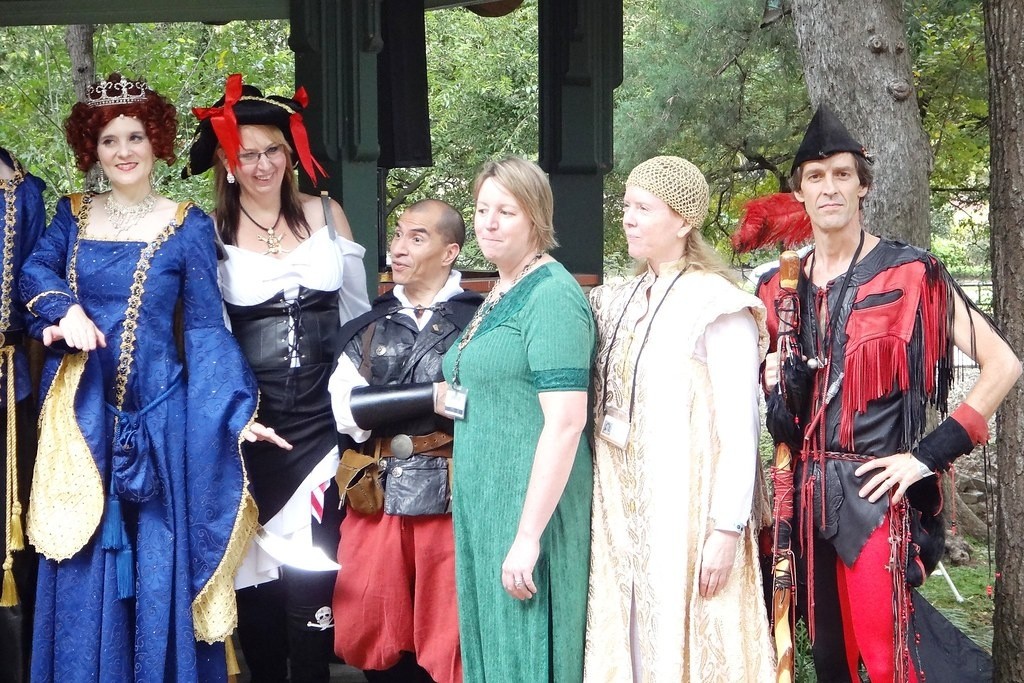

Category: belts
[352, 428, 454, 459]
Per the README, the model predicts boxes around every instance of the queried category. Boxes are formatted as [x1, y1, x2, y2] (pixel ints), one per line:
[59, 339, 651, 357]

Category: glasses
[234, 143, 283, 166]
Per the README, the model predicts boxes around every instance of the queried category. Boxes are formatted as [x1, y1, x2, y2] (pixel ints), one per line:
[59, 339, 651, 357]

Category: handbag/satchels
[339, 452, 383, 515]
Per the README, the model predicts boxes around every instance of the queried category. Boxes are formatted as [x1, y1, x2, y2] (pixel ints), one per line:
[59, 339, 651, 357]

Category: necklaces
[808, 229, 866, 367]
[240, 201, 291, 256]
[103, 190, 158, 236]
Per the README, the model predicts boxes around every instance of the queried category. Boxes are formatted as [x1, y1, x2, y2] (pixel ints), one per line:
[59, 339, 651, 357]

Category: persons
[0, 140, 48, 683]
[586, 100, 1023, 683]
[181, 74, 600, 683]
[18, 70, 293, 683]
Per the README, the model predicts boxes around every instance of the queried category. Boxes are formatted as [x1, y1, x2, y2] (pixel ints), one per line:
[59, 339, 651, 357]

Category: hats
[790, 105, 874, 177]
[191, 75, 327, 187]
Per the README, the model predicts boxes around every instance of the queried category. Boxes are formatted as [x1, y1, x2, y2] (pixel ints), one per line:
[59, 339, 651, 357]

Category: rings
[515, 580, 523, 590]
[266, 427, 275, 432]
